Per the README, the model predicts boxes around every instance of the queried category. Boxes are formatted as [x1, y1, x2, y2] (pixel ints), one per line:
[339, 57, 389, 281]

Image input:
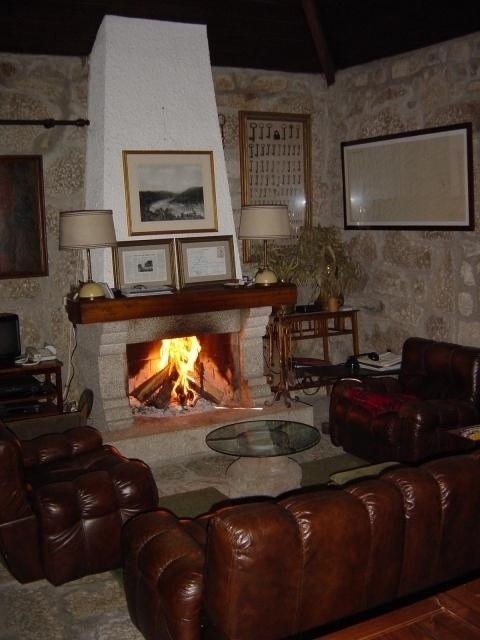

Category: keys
[248, 121, 306, 206]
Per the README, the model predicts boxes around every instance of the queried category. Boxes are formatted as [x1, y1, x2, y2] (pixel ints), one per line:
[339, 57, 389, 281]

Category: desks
[264, 310, 360, 407]
[315, 577, 480, 640]
[0, 357, 63, 422]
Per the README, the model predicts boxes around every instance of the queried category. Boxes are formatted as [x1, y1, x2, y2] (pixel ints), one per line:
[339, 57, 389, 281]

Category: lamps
[238, 204, 289, 284]
[59, 209, 117, 298]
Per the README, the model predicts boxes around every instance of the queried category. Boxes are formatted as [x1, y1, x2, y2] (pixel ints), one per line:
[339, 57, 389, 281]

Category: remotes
[368, 352, 380, 361]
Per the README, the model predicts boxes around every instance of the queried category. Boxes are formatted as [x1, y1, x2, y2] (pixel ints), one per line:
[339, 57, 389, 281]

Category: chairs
[329, 337, 480, 467]
[0, 422, 159, 588]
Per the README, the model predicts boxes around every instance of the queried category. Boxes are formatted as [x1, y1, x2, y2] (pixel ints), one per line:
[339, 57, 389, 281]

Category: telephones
[24, 345, 56, 362]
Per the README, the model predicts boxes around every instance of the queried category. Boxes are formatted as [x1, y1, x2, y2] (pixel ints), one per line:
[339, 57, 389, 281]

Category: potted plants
[297, 222, 366, 312]
[266, 247, 301, 315]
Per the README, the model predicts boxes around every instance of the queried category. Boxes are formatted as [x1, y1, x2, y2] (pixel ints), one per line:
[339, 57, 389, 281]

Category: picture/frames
[112, 238, 177, 291]
[340, 122, 475, 231]
[121, 149, 218, 237]
[175, 234, 239, 290]
[239, 110, 313, 264]
[0, 155, 49, 279]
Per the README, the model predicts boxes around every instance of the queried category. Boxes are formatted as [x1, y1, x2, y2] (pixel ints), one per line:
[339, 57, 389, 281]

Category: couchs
[121, 461, 480, 640]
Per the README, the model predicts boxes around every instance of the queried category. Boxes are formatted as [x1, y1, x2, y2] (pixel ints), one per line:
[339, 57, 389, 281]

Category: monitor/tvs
[0, 313, 21, 367]
